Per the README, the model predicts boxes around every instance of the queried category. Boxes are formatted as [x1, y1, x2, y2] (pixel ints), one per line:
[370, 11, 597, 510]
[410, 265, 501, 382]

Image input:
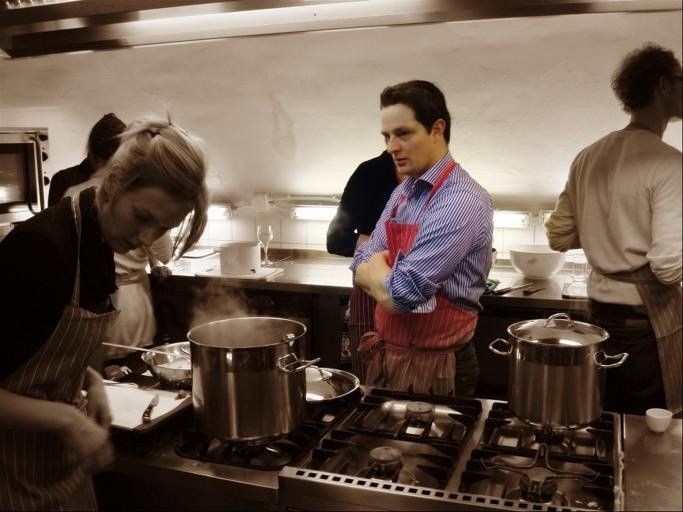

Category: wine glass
[257, 225, 273, 265]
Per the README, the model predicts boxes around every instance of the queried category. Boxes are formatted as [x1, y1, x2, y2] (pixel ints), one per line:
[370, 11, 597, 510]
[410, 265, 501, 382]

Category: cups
[646, 407, 673, 431]
[219, 242, 262, 274]
[571, 255, 589, 280]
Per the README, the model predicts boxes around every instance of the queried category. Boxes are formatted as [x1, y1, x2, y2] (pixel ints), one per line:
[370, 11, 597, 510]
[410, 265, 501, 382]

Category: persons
[46, 111, 177, 381]
[542, 39, 682, 419]
[0, 116, 211, 510]
[346, 79, 495, 398]
[322, 147, 409, 385]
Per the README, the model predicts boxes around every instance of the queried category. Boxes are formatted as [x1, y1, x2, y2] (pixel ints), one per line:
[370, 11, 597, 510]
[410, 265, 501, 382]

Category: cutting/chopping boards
[195, 264, 284, 282]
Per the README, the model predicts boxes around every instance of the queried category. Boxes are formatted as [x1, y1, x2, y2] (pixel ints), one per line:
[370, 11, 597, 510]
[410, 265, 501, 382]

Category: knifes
[141, 393, 159, 422]
[496, 282, 533, 295]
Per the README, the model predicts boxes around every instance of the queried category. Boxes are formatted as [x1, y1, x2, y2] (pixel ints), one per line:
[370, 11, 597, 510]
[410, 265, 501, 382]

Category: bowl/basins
[300, 364, 362, 406]
[141, 341, 193, 384]
[506, 245, 566, 279]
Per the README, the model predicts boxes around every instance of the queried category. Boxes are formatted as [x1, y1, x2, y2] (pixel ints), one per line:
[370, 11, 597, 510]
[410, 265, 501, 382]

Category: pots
[488, 314, 629, 431]
[186, 311, 323, 446]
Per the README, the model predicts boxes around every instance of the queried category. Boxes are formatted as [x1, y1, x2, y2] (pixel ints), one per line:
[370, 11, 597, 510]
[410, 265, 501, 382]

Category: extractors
[0, 0, 681, 60]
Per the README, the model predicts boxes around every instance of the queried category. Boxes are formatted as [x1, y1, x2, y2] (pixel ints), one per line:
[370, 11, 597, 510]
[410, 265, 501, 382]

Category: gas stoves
[142, 384, 622, 510]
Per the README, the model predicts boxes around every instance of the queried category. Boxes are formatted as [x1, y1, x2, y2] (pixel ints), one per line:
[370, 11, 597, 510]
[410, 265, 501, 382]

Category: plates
[564, 278, 590, 300]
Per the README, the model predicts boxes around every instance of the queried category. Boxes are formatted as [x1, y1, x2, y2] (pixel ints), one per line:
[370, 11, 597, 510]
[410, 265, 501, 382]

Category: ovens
[0, 126, 52, 223]
